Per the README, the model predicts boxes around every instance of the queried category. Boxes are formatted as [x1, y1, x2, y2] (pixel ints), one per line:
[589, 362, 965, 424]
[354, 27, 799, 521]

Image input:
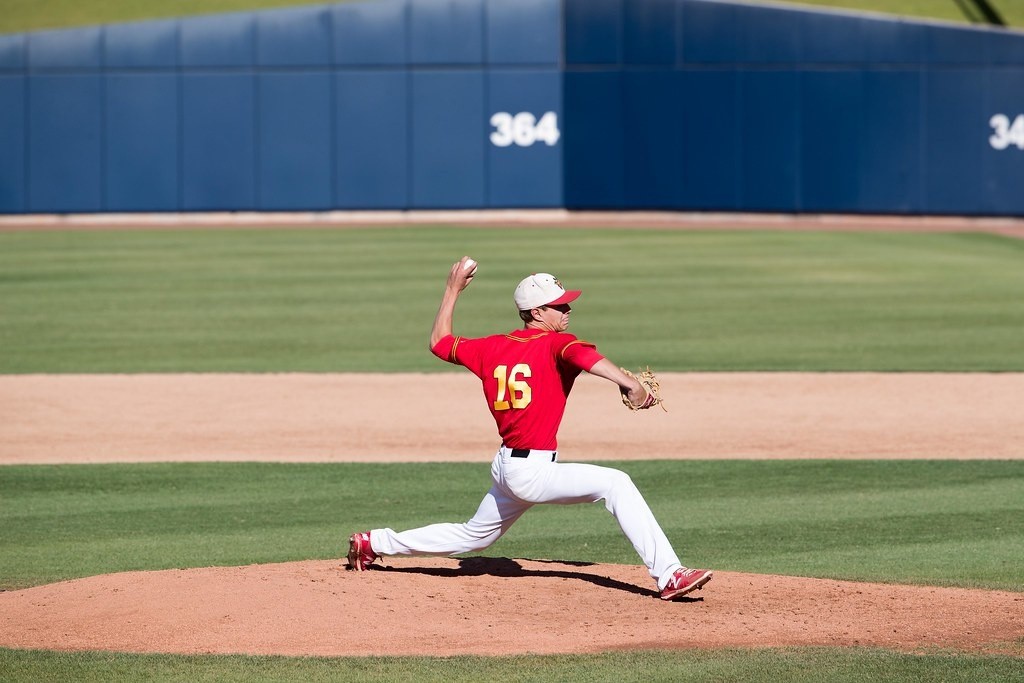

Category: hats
[514, 272, 582, 310]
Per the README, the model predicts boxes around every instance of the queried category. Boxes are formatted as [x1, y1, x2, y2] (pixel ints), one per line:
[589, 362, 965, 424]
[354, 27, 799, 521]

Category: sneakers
[661, 567, 714, 599]
[346, 531, 383, 572]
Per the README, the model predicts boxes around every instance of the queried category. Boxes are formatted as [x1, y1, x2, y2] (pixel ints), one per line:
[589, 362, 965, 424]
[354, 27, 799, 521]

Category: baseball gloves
[618, 364, 664, 412]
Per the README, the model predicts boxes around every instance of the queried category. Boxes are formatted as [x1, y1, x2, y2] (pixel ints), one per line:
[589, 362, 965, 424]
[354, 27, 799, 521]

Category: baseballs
[464, 259, 478, 276]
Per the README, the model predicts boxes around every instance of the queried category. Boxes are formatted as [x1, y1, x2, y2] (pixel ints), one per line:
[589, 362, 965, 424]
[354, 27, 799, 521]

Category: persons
[344, 255, 713, 600]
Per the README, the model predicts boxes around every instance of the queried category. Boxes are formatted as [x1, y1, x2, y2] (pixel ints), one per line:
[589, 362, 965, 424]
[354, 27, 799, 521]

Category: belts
[511, 449, 556, 462]
